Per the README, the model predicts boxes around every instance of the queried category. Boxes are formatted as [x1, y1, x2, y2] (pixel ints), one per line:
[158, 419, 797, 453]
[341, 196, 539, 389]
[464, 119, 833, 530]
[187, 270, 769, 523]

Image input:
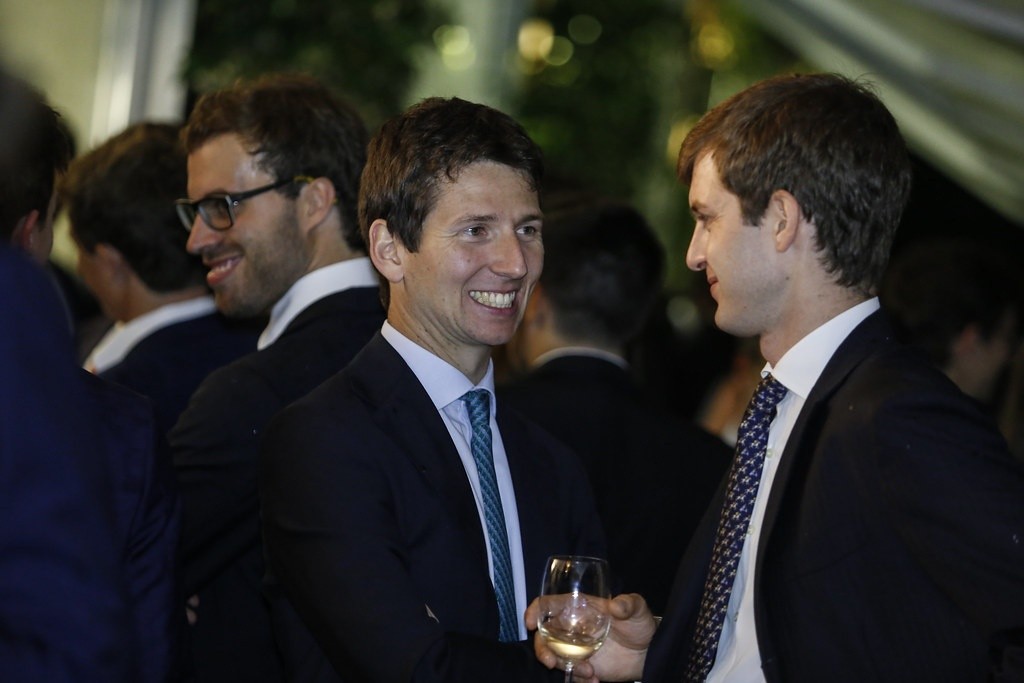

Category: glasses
[175, 174, 345, 231]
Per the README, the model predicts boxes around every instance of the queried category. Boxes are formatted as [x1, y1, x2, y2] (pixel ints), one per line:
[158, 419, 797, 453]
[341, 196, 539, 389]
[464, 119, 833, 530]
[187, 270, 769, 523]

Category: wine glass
[536, 556, 612, 683]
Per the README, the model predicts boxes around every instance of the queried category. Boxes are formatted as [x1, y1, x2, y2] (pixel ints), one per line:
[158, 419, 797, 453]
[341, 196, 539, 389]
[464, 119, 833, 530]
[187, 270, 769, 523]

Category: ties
[685, 373, 788, 683]
[457, 388, 519, 642]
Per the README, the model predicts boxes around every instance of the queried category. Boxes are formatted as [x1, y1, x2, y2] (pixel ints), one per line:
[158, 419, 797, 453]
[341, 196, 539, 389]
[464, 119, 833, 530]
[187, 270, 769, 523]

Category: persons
[888, 202, 1024, 466]
[62, 120, 269, 434]
[0, 68, 184, 683]
[258, 96, 615, 683]
[629, 254, 761, 443]
[492, 200, 735, 683]
[526, 74, 1024, 683]
[163, 75, 389, 683]
